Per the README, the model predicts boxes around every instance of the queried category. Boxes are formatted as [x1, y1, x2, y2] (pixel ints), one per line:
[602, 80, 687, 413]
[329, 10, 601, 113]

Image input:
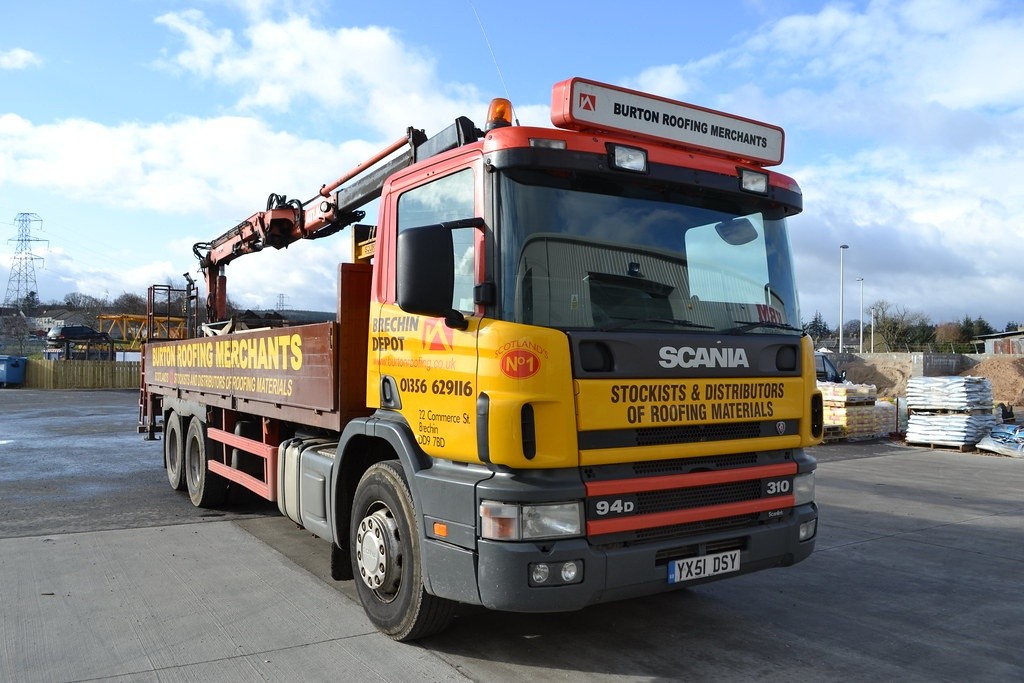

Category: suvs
[45, 324, 112, 348]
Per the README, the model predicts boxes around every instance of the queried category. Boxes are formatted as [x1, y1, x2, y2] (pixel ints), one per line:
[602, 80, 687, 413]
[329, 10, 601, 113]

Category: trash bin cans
[0, 355, 27, 388]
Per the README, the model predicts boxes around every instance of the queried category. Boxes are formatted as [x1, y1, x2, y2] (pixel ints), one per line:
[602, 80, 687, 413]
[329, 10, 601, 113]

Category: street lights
[871, 306, 875, 353]
[839, 243, 849, 353]
[856, 277, 863, 353]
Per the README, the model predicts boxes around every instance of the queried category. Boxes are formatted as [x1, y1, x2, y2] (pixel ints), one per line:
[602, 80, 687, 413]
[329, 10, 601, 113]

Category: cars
[814, 354, 846, 383]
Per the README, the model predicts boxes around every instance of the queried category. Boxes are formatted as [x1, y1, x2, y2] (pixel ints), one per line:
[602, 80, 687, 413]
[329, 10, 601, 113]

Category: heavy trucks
[137, 77, 825, 642]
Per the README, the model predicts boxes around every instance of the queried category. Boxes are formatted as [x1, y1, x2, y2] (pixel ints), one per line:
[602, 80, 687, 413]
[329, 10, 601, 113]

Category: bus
[0, 305, 28, 320]
